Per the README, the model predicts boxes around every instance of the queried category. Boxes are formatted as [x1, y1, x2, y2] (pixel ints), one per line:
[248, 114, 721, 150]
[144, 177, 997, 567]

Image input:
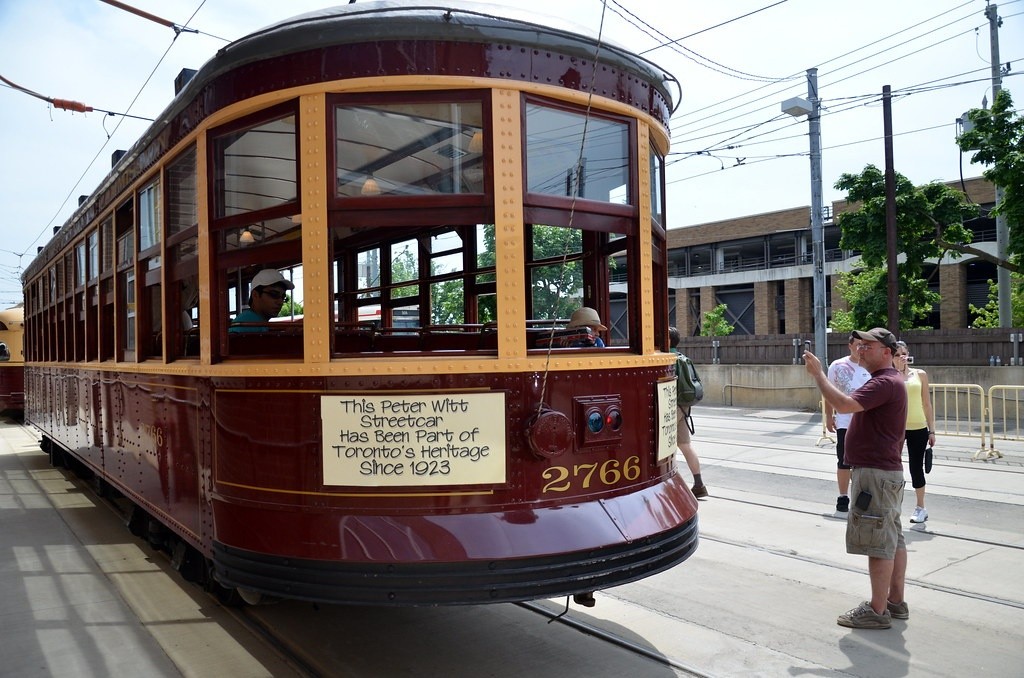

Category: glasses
[257, 288, 289, 303]
[855, 344, 891, 353]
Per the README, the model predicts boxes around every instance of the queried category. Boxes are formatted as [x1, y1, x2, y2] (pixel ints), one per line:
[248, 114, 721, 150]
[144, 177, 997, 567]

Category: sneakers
[886, 598, 909, 619]
[836, 495, 850, 512]
[837, 601, 894, 629]
[690, 485, 709, 499]
[910, 506, 928, 524]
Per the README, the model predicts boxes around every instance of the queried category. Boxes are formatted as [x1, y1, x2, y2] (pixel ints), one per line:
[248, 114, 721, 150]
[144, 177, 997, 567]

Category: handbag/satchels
[924, 444, 933, 473]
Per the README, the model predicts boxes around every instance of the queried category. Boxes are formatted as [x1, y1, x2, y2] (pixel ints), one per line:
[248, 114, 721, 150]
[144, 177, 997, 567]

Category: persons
[566, 307, 608, 349]
[823, 331, 872, 511]
[229, 269, 296, 332]
[668, 327, 710, 499]
[888, 340, 936, 523]
[178, 277, 199, 330]
[804, 327, 910, 629]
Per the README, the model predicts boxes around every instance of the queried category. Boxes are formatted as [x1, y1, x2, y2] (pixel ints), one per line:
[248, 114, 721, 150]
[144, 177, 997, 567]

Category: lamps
[360, 170, 382, 195]
[239, 226, 255, 242]
[468, 132, 482, 154]
[291, 214, 301, 225]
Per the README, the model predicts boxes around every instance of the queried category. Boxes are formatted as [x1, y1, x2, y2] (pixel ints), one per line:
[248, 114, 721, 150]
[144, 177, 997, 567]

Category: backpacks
[675, 353, 704, 435]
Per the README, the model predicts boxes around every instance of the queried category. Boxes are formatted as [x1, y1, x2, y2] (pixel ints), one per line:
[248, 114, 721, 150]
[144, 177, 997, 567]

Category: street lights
[782, 66, 829, 377]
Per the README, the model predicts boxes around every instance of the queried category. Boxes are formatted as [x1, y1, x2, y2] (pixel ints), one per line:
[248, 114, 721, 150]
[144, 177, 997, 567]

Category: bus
[22, 0, 684, 618]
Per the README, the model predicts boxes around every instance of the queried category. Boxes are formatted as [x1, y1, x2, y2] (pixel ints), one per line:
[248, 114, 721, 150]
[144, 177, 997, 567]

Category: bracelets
[928, 431, 936, 435]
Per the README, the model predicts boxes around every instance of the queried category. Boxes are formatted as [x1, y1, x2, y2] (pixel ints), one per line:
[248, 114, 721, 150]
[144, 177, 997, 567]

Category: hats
[850, 327, 899, 356]
[565, 307, 608, 332]
[249, 269, 295, 295]
